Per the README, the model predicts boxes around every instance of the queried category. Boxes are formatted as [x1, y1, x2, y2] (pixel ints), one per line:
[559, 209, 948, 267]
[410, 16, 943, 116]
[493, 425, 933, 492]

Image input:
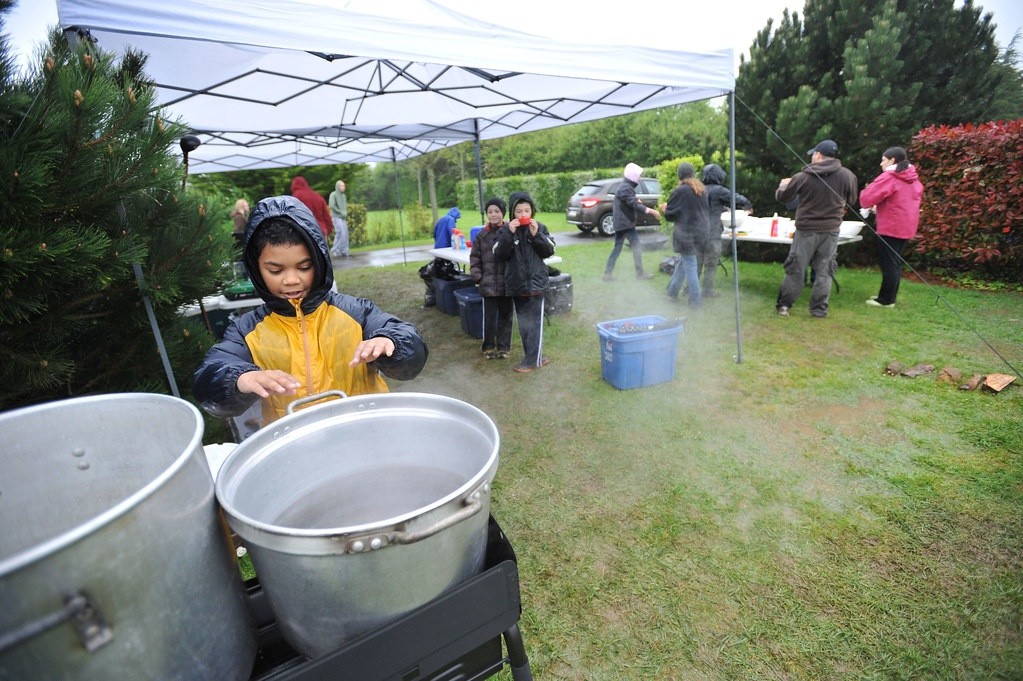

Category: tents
[55, 0, 743, 397]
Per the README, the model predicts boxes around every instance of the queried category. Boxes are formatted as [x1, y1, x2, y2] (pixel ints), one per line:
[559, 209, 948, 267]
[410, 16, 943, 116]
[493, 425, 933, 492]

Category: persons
[290, 176, 333, 241]
[859, 147, 924, 309]
[328, 180, 348, 257]
[660, 162, 712, 310]
[775, 139, 858, 317]
[683, 164, 752, 298]
[191, 195, 428, 427]
[231, 199, 249, 231]
[469, 198, 514, 360]
[492, 192, 556, 372]
[433, 208, 460, 249]
[602, 162, 660, 281]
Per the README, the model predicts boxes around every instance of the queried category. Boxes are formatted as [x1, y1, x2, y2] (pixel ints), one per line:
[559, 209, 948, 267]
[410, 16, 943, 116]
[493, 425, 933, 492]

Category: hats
[807, 140, 838, 157]
[485, 197, 507, 218]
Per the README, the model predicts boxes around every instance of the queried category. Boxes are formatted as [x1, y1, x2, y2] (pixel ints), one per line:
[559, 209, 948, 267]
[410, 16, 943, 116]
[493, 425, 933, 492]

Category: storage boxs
[545, 272, 573, 315]
[432, 271, 476, 317]
[596, 314, 683, 391]
[451, 286, 498, 339]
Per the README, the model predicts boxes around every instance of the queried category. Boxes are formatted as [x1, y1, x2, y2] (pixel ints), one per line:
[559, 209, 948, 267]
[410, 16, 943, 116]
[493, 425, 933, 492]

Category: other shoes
[602, 274, 619, 281]
[541, 357, 549, 365]
[811, 309, 827, 318]
[869, 295, 881, 300]
[866, 300, 895, 309]
[638, 273, 654, 280]
[778, 305, 791, 316]
[515, 364, 533, 372]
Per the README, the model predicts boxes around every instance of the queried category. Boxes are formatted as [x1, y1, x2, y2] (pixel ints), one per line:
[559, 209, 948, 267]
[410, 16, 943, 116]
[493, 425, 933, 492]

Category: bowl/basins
[839, 221, 866, 238]
[721, 210, 748, 228]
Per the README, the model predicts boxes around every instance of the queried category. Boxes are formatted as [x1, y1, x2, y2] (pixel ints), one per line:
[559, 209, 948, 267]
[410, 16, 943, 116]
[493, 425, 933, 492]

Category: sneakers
[482, 349, 495, 361]
[496, 349, 509, 359]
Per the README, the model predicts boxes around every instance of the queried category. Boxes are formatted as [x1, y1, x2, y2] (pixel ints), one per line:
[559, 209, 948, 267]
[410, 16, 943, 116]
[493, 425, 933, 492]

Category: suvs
[565, 177, 663, 239]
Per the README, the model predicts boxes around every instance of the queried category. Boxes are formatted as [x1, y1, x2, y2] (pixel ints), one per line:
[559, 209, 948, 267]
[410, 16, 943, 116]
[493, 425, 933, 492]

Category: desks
[427, 245, 562, 326]
[174, 276, 338, 316]
[716, 225, 864, 295]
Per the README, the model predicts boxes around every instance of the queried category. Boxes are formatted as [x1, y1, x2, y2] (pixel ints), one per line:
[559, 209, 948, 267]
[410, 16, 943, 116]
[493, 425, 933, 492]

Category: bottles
[770, 213, 778, 237]
[451, 228, 465, 251]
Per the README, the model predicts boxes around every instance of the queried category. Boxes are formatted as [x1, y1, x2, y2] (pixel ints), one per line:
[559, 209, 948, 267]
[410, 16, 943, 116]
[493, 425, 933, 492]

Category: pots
[215, 390, 500, 661]
[0, 392, 256, 681]
